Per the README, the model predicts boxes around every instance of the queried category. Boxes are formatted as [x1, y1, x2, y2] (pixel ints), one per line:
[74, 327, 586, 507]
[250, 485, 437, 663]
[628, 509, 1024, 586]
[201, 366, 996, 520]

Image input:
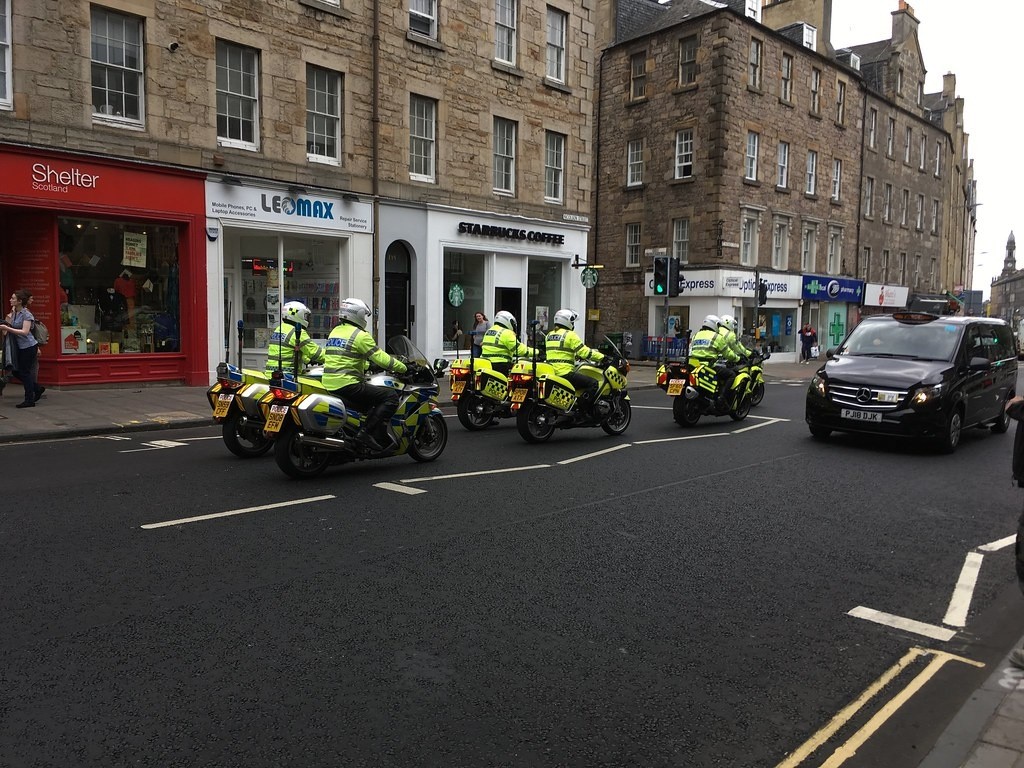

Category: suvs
[804, 312, 1016, 453]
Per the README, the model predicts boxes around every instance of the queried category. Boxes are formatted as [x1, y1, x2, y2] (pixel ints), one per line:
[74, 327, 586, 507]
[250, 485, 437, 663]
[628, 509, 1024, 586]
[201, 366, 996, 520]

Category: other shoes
[16, 401, 35, 408]
[33, 387, 45, 402]
[806, 362, 809, 364]
[801, 360, 805, 363]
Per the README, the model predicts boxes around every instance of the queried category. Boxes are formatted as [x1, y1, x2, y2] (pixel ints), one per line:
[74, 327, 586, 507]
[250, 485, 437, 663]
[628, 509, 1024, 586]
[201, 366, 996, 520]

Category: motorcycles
[506, 318, 634, 444]
[261, 281, 452, 481]
[656, 350, 755, 429]
[207, 298, 297, 459]
[448, 318, 525, 434]
[737, 335, 773, 408]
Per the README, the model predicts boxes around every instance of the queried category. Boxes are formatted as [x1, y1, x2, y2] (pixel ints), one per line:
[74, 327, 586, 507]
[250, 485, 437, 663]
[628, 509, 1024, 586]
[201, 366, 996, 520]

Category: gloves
[749, 352, 757, 359]
[736, 356, 746, 366]
[539, 349, 545, 357]
[602, 354, 613, 366]
[404, 364, 418, 376]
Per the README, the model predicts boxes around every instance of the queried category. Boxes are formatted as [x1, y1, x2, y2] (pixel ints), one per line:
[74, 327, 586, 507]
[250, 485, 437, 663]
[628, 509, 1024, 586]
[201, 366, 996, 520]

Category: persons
[1005, 389, 1024, 669]
[543, 310, 615, 422]
[0, 289, 47, 408]
[266, 299, 323, 381]
[97, 288, 129, 332]
[674, 319, 681, 339]
[449, 320, 463, 341]
[324, 296, 416, 448]
[799, 322, 818, 365]
[874, 330, 897, 352]
[482, 311, 539, 427]
[720, 315, 760, 369]
[688, 315, 749, 411]
[472, 312, 492, 359]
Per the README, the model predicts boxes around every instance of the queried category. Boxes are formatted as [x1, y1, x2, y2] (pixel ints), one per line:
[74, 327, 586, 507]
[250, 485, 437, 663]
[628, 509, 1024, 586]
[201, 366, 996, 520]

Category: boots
[717, 377, 734, 410]
[354, 415, 383, 450]
[573, 391, 596, 414]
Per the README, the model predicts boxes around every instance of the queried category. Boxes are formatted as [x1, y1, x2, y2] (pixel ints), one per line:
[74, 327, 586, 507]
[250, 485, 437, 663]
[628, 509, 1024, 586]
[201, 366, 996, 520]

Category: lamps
[342, 194, 360, 203]
[288, 186, 308, 195]
[222, 176, 243, 186]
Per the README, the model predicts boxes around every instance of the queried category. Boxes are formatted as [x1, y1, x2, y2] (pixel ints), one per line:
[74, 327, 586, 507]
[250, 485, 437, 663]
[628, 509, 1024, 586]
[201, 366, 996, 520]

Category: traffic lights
[672, 259, 685, 297]
[759, 282, 767, 306]
[652, 257, 671, 298]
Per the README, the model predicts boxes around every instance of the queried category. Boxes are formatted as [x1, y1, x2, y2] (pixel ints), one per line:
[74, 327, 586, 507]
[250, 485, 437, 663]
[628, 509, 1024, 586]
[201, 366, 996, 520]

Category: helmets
[719, 315, 738, 331]
[702, 315, 721, 334]
[339, 297, 371, 330]
[553, 310, 575, 331]
[281, 301, 311, 328]
[494, 311, 517, 331]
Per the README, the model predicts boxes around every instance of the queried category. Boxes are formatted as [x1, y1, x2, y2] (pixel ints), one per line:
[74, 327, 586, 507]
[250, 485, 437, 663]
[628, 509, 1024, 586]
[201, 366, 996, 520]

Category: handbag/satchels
[811, 346, 819, 357]
[31, 319, 48, 345]
